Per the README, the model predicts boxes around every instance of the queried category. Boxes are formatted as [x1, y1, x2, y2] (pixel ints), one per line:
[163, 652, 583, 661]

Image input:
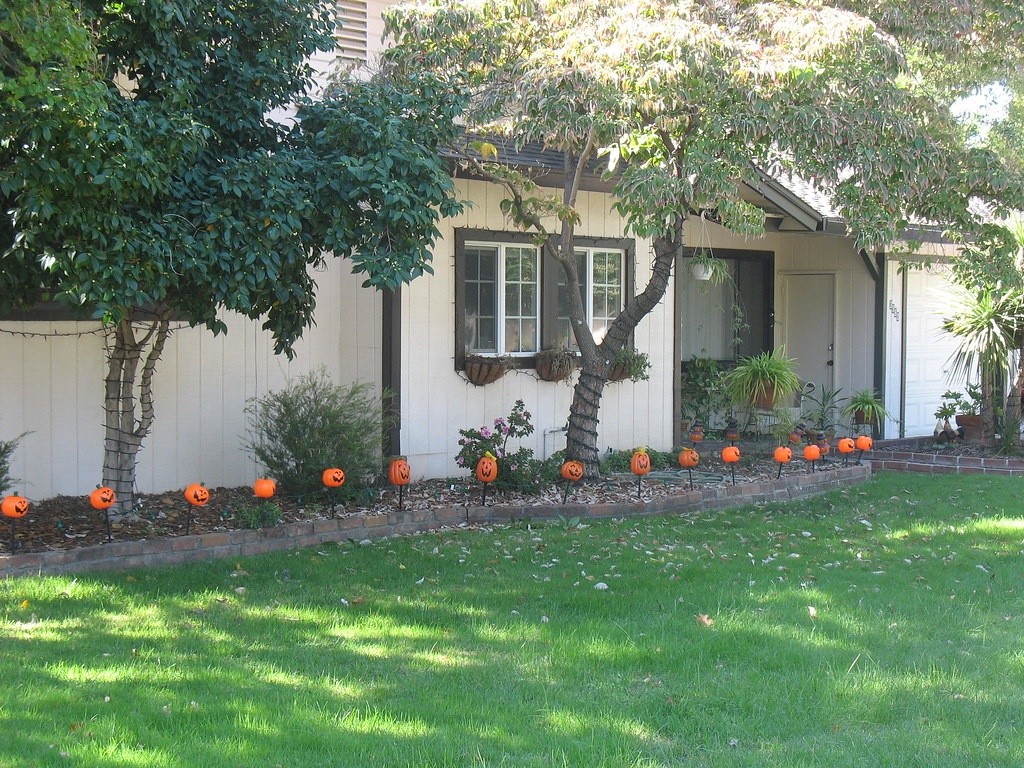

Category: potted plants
[801, 383, 848, 445]
[934, 382, 1005, 444]
[717, 344, 801, 411]
[465, 357, 510, 386]
[533, 343, 578, 382]
[686, 248, 751, 348]
[841, 387, 902, 435]
[607, 346, 653, 384]
